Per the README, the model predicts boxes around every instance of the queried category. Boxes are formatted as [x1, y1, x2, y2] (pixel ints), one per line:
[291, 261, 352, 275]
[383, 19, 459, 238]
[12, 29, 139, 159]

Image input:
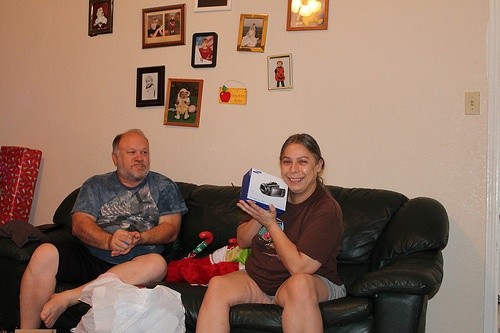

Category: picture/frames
[191, 32, 218, 68]
[237, 14, 269, 53]
[194, 0, 231, 12]
[162, 78, 204, 127]
[286, 0, 329, 31]
[88, 0, 114, 36]
[135, 65, 165, 107]
[141, 3, 185, 49]
[267, 53, 293, 91]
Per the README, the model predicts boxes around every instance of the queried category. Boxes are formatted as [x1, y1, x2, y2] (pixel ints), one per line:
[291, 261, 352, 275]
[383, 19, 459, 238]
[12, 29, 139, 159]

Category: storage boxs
[0, 145, 42, 225]
[240, 167, 289, 217]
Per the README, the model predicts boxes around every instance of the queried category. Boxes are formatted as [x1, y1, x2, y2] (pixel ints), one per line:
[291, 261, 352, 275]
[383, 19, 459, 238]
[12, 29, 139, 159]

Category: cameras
[260, 182, 285, 197]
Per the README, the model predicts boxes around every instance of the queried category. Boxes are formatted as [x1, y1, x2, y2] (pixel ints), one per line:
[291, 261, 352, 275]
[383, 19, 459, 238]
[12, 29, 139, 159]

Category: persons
[165, 16, 180, 36]
[241, 23, 257, 47]
[199, 39, 212, 61]
[147, 17, 163, 38]
[19, 130, 188, 329]
[196, 133, 346, 333]
[275, 61, 285, 87]
[175, 89, 190, 120]
[146, 76, 156, 96]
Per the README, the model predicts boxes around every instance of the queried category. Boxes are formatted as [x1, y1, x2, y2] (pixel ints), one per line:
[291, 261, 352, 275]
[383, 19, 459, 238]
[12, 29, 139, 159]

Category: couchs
[0, 182, 449, 333]
